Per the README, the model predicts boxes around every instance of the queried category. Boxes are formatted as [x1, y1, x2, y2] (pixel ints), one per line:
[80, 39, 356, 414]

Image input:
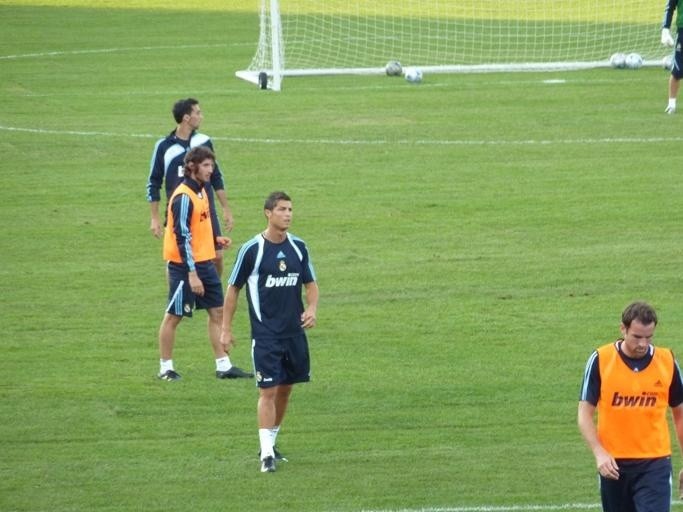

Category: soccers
[611, 53, 624, 67]
[661, 55, 674, 69]
[406, 70, 422, 81]
[625, 53, 642, 68]
[386, 60, 402, 75]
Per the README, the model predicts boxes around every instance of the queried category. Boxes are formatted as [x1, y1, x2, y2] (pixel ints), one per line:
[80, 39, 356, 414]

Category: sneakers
[216, 366, 254, 378]
[665, 107, 676, 114]
[160, 370, 182, 381]
[259, 446, 288, 472]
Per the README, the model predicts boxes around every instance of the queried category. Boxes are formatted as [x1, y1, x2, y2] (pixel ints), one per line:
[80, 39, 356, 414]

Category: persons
[156, 145, 254, 381]
[576, 300, 681, 511]
[660, 1, 682, 114]
[144, 98, 235, 279]
[219, 191, 318, 473]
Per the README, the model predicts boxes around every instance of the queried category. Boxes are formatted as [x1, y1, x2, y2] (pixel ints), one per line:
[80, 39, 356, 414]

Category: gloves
[661, 28, 674, 47]
